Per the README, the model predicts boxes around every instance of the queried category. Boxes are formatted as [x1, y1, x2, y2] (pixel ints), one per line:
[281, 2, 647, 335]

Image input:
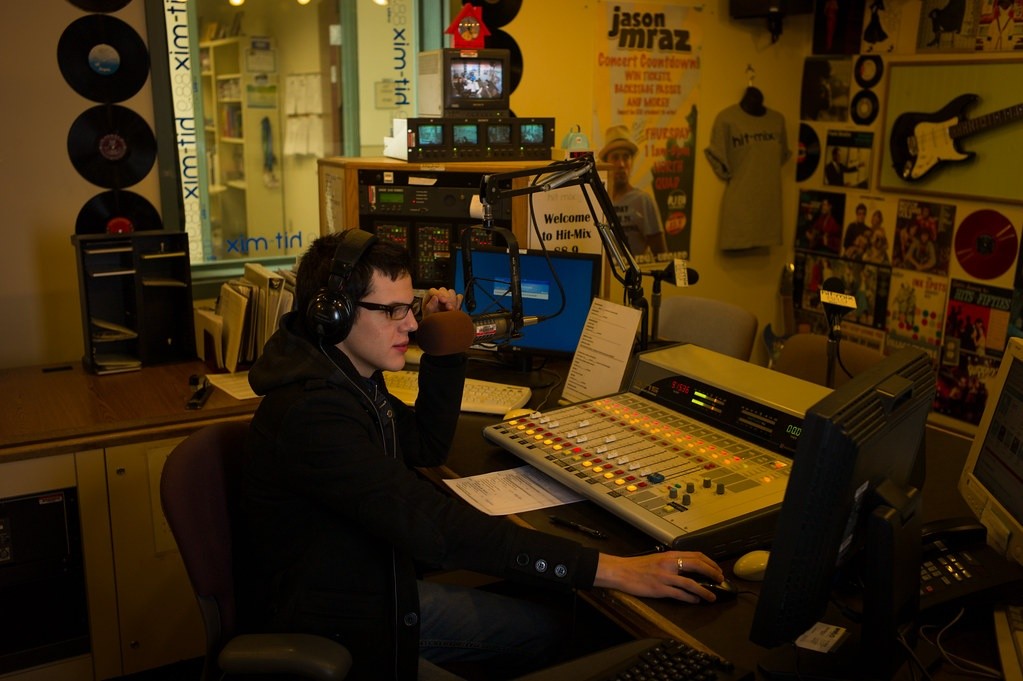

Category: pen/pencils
[548, 516, 608, 539]
[42, 366, 75, 372]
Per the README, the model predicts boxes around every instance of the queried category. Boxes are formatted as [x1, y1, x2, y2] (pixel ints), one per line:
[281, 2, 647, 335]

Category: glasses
[361, 297, 423, 321]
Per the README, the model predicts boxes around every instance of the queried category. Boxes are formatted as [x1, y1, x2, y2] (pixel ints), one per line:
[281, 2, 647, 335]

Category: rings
[678, 558, 682, 568]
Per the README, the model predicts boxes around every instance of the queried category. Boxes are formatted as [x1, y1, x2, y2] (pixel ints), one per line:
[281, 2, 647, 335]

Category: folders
[245, 262, 286, 344]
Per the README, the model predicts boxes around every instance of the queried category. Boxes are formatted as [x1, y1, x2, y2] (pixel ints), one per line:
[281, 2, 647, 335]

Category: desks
[400, 349, 1006, 681]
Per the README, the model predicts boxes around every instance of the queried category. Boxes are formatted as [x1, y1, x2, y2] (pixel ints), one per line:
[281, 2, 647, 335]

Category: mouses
[503, 407, 541, 422]
[654, 569, 738, 606]
[733, 550, 771, 580]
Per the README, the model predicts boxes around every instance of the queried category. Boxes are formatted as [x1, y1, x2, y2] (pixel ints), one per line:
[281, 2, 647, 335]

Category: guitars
[889, 93, 1023, 183]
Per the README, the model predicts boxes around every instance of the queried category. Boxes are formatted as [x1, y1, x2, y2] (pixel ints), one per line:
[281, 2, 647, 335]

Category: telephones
[873, 514, 1021, 622]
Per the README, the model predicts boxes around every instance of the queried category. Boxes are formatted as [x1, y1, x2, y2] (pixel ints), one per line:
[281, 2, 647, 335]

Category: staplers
[184, 373, 214, 409]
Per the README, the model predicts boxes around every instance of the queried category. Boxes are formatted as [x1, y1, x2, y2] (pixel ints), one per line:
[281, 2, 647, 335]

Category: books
[206, 150, 217, 186]
[217, 262, 298, 374]
[222, 104, 242, 138]
[207, 11, 243, 39]
[91, 318, 142, 375]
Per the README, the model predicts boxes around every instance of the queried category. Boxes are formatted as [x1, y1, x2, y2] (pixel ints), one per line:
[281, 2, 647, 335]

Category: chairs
[632, 296, 887, 389]
[158, 421, 389, 681]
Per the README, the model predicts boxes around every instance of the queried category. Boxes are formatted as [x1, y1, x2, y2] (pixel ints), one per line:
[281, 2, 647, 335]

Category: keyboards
[586, 637, 756, 681]
[383, 370, 532, 413]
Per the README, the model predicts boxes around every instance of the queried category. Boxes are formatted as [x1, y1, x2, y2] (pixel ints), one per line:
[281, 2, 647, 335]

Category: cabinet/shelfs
[1, 352, 273, 680]
[198, 35, 287, 259]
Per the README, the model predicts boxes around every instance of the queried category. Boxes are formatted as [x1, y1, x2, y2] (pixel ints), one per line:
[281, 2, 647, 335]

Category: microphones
[417, 311, 546, 355]
[820, 277, 857, 342]
[641, 258, 699, 287]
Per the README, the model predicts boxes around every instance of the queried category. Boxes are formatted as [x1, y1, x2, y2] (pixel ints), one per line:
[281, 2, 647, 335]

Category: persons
[844, 203, 889, 264]
[927, 0, 966, 46]
[806, 199, 839, 250]
[225, 228, 724, 681]
[950, 377, 966, 399]
[825, 148, 865, 186]
[598, 125, 665, 262]
[948, 304, 986, 356]
[863, 0, 894, 53]
[901, 206, 936, 271]
[809, 257, 870, 322]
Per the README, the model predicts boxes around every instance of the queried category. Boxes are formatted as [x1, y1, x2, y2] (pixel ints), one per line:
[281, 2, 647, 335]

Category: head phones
[306, 228, 375, 346]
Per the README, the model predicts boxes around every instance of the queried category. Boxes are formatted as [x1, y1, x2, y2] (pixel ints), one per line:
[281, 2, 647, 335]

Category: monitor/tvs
[750, 347, 941, 681]
[407, 49, 555, 162]
[449, 244, 602, 388]
[957, 336, 1023, 564]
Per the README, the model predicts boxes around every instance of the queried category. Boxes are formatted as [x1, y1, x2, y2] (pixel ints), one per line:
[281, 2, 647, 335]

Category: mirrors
[147, 0, 360, 282]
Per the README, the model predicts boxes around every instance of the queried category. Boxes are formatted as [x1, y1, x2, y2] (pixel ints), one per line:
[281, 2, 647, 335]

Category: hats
[598, 123, 639, 162]
